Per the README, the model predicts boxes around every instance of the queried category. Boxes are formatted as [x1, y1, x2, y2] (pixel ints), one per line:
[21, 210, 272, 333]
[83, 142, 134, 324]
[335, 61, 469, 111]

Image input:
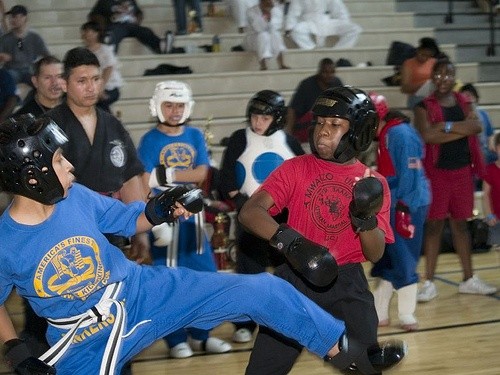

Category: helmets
[149, 80, 195, 124]
[366, 92, 389, 121]
[308, 87, 379, 162]
[246, 90, 288, 137]
[0, 113, 69, 206]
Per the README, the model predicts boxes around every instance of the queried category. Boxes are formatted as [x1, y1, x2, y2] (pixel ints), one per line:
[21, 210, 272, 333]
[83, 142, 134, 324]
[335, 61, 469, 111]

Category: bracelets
[486, 214, 494, 220]
[445, 121, 453, 132]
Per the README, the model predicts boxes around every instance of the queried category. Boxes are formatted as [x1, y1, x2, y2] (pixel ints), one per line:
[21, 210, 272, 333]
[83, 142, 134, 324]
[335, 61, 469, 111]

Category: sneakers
[456, 273, 497, 295]
[416, 279, 437, 303]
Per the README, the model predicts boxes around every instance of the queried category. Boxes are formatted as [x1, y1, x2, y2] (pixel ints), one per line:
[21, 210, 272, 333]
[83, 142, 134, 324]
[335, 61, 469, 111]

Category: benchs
[1, 0, 500, 220]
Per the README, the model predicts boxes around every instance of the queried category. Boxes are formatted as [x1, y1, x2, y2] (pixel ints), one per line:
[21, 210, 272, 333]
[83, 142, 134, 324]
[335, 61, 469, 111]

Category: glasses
[431, 73, 455, 81]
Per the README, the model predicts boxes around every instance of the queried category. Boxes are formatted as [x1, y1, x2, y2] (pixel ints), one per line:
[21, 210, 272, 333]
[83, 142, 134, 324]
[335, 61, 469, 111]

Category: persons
[24, 47, 151, 375]
[0, 0, 500, 371]
[283, 57, 344, 138]
[402, 36, 442, 95]
[0, 113, 404, 375]
[136, 81, 232, 359]
[82, 21, 122, 107]
[365, 89, 430, 330]
[412, 57, 497, 302]
[222, 90, 306, 344]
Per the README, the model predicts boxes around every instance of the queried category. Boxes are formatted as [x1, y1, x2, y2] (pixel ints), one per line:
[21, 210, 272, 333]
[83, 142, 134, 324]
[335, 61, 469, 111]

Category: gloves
[152, 222, 173, 247]
[348, 176, 384, 231]
[231, 192, 249, 212]
[395, 200, 414, 240]
[270, 223, 338, 287]
[149, 166, 176, 189]
[2, 338, 56, 375]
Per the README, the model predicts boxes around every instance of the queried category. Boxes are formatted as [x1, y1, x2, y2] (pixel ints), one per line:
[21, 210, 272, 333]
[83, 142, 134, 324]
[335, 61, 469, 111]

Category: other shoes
[324, 335, 404, 375]
[399, 313, 418, 331]
[233, 329, 251, 342]
[170, 342, 193, 358]
[164, 30, 173, 54]
[205, 337, 231, 352]
[377, 312, 390, 327]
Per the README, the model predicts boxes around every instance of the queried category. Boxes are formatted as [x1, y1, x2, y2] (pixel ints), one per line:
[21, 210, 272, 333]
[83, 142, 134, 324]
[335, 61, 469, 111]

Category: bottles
[212, 38, 220, 53]
